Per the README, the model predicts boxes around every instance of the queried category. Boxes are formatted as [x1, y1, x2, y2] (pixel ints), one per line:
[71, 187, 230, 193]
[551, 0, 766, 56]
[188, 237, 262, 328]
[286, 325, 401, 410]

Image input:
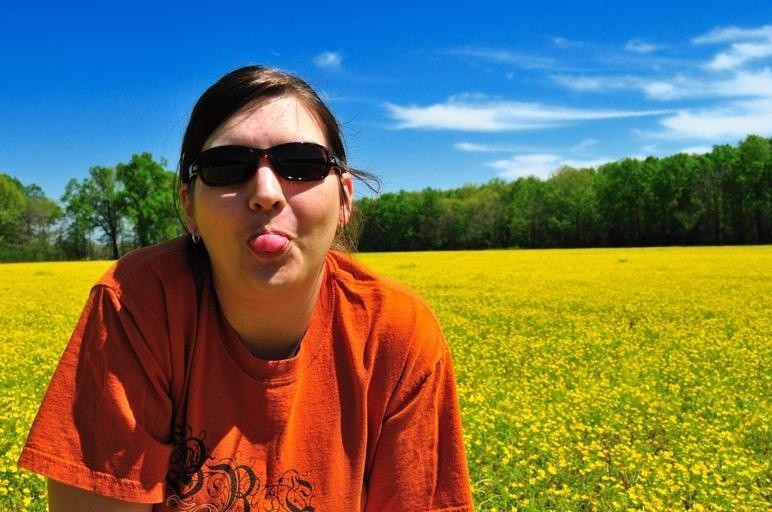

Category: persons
[16, 62, 473, 512]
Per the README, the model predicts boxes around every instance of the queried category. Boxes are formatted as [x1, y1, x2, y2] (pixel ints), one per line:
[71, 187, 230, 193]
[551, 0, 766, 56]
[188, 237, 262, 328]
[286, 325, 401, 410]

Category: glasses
[188, 142, 340, 188]
